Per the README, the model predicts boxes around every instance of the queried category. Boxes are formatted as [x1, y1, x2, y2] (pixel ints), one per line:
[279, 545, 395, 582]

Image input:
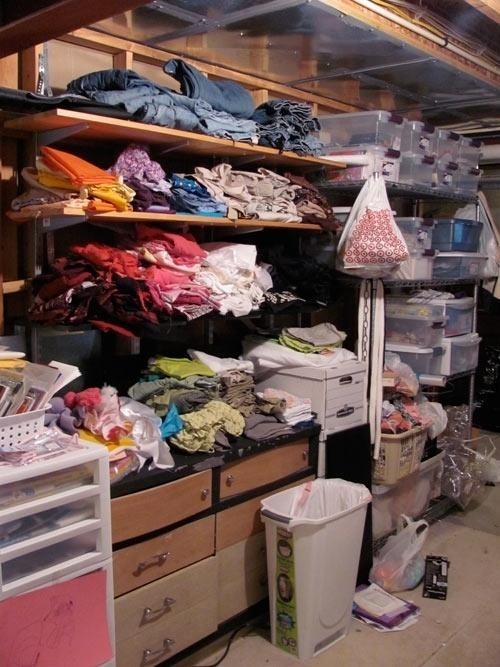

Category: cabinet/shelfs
[242, 342, 367, 440]
[110, 425, 322, 667]
[0, 423, 115, 666]
[324, 150, 483, 563]
[1, 109, 346, 300]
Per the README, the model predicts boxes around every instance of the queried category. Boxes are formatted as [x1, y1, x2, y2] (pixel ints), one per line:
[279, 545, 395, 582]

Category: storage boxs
[433, 129, 465, 148]
[402, 121, 439, 149]
[383, 254, 432, 281]
[424, 219, 482, 255]
[431, 157, 457, 193]
[433, 251, 483, 281]
[439, 336, 482, 377]
[322, 149, 399, 187]
[397, 151, 432, 188]
[384, 298, 448, 345]
[323, 111, 408, 147]
[380, 343, 443, 380]
[430, 297, 475, 337]
[370, 449, 452, 539]
[393, 218, 436, 252]
[458, 136, 484, 161]
[457, 163, 482, 195]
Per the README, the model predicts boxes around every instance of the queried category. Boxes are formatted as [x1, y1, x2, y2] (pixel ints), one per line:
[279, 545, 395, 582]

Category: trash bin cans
[260, 478, 373, 661]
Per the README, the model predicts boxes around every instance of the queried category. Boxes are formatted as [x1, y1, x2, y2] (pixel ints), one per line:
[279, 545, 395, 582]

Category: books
[0, 358, 82, 417]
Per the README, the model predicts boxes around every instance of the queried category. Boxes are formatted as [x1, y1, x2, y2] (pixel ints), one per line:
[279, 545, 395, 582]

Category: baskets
[372, 419, 434, 486]
[0, 393, 52, 454]
